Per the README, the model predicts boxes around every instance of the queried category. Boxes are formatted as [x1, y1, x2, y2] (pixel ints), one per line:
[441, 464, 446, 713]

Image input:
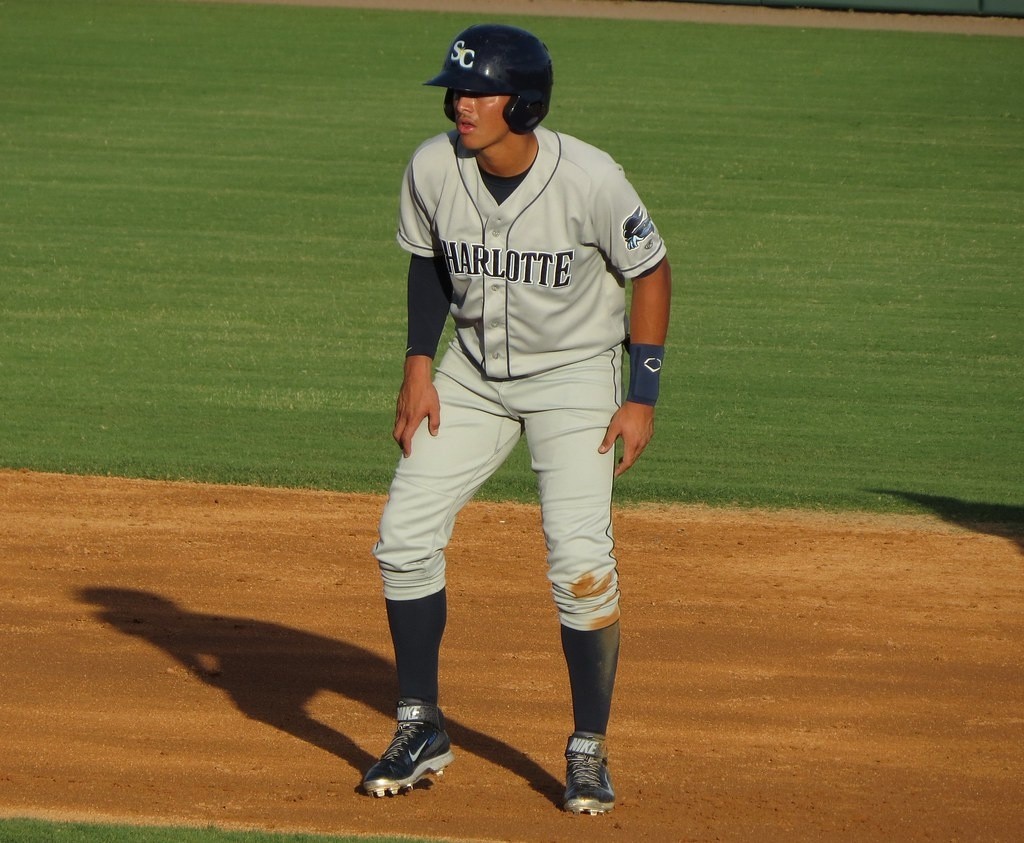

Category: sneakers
[362, 697, 455, 797]
[563, 731, 615, 816]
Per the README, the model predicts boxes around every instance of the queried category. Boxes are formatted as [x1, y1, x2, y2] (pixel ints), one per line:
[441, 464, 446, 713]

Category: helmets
[421, 24, 553, 134]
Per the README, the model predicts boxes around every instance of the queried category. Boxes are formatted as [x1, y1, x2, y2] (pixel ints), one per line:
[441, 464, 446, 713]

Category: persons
[362, 26, 671, 813]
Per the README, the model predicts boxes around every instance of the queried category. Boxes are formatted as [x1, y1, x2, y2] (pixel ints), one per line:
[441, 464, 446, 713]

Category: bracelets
[627, 344, 664, 406]
